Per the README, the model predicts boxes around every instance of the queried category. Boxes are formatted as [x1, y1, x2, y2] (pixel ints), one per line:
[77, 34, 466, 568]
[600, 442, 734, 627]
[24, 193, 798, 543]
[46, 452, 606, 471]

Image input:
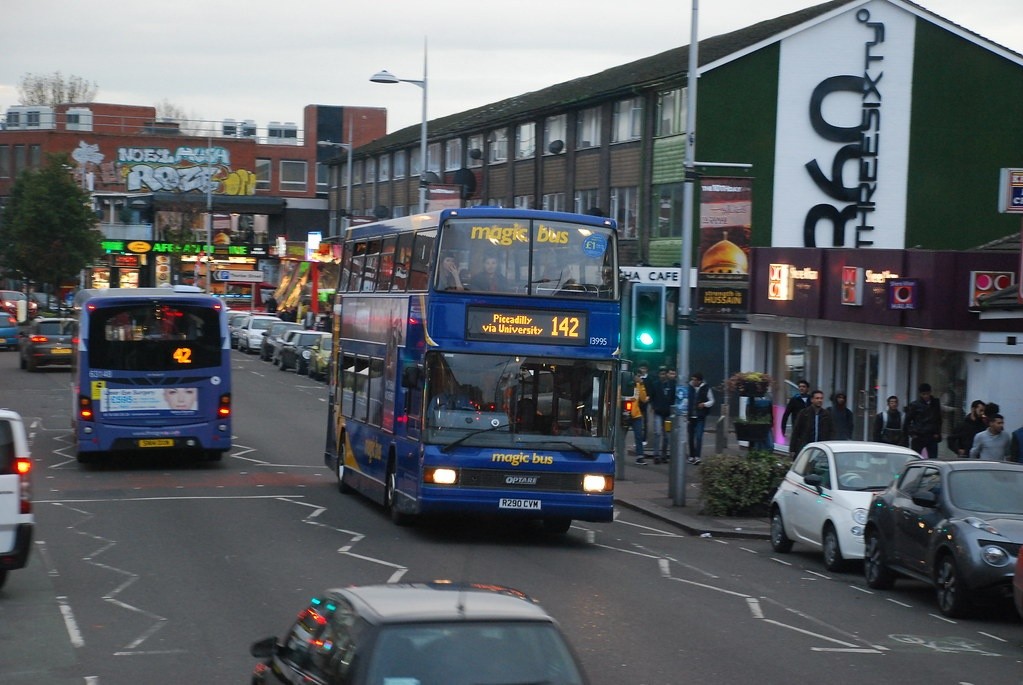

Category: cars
[0, 290, 38, 321]
[769, 441, 923, 575]
[278, 330, 328, 375]
[259, 322, 303, 362]
[0, 312, 20, 352]
[863, 457, 1023, 620]
[225, 310, 249, 350]
[308, 333, 333, 381]
[23, 291, 75, 316]
[250, 579, 593, 684]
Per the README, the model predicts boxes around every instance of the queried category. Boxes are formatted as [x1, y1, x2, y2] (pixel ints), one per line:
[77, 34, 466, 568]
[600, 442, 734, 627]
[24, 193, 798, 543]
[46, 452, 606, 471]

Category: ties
[451, 395, 456, 410]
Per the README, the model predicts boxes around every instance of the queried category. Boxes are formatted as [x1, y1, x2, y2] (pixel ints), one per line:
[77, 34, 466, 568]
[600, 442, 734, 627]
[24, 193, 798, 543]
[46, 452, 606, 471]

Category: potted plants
[732, 418, 774, 445]
[731, 372, 772, 399]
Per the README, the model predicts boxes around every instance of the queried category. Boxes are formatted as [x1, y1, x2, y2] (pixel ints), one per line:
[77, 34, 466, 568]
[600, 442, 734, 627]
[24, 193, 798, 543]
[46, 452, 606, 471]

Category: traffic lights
[631, 281, 667, 353]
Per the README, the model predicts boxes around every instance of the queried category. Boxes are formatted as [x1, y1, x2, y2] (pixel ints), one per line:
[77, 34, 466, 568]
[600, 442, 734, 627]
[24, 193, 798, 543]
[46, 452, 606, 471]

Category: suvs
[19, 315, 78, 373]
[0, 409, 34, 592]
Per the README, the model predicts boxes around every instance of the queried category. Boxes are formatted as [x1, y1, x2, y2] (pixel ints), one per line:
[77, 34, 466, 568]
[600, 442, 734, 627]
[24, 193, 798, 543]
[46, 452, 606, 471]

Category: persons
[436, 252, 464, 290]
[469, 253, 509, 291]
[780, 378, 1023, 474]
[280, 306, 333, 334]
[427, 370, 477, 428]
[538, 247, 575, 295]
[621, 362, 716, 464]
[263, 293, 278, 316]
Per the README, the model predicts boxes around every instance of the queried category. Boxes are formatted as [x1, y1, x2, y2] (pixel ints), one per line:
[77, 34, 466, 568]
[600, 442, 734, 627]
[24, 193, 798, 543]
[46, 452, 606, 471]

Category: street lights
[316, 113, 355, 217]
[367, 35, 431, 215]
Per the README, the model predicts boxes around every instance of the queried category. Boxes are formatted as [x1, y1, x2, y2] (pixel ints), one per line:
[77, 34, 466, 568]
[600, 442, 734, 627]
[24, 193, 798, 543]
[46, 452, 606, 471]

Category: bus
[323, 207, 635, 543]
[72, 284, 233, 465]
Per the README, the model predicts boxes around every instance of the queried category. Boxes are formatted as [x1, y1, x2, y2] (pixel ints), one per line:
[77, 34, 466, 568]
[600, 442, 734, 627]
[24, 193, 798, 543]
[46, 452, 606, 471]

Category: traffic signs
[212, 270, 263, 282]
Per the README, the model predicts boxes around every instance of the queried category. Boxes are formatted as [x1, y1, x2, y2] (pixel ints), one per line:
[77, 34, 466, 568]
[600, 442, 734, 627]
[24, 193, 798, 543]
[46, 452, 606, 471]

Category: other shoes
[636, 458, 647, 465]
[654, 457, 662, 464]
[688, 456, 694, 463]
[662, 454, 670, 461]
[693, 459, 701, 465]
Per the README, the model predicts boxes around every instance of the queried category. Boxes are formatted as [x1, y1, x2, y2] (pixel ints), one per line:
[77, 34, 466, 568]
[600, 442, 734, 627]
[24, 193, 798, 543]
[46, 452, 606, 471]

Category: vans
[237, 315, 283, 355]
